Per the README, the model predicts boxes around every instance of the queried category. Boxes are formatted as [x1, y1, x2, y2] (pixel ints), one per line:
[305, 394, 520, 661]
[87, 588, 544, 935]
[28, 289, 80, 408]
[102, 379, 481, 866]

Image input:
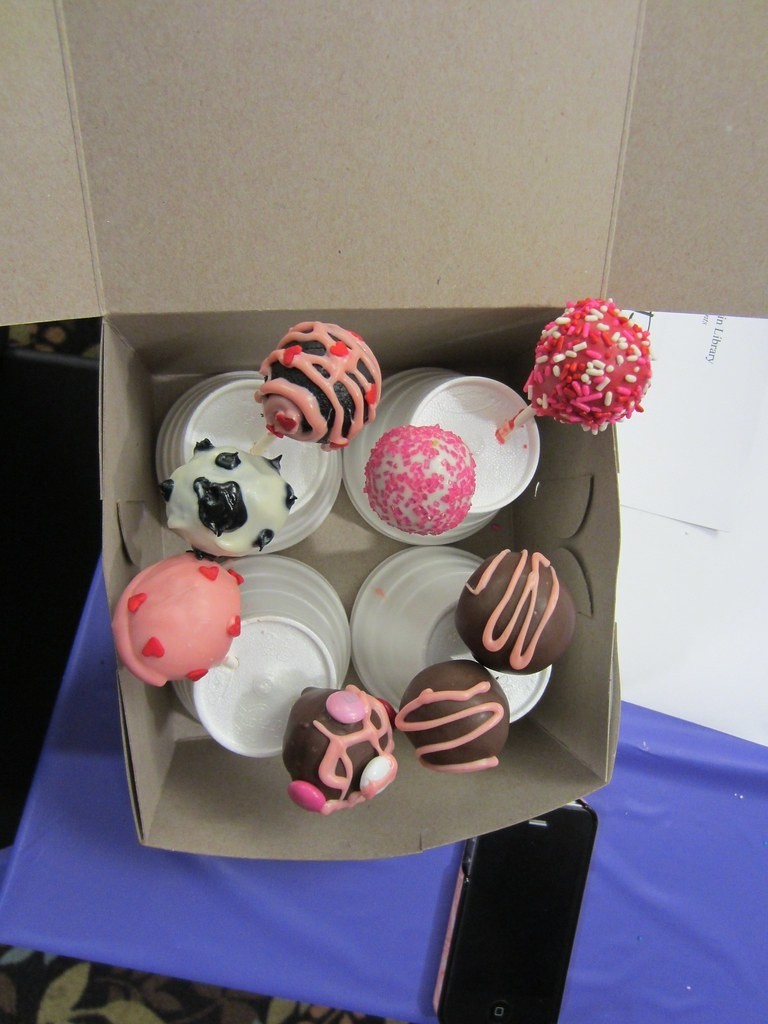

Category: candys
[112, 299, 655, 814]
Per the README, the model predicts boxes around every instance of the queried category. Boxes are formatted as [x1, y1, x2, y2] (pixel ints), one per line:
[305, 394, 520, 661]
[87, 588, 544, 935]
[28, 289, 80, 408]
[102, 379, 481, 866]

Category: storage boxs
[0, 0, 768, 866]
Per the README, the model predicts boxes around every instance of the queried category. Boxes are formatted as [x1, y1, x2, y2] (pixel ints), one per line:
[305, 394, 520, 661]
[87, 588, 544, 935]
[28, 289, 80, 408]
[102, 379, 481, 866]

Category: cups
[151, 357, 553, 763]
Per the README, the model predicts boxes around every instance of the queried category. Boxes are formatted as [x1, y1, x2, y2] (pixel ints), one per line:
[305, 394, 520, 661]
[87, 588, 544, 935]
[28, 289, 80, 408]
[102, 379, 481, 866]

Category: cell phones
[434, 796, 599, 1024]
